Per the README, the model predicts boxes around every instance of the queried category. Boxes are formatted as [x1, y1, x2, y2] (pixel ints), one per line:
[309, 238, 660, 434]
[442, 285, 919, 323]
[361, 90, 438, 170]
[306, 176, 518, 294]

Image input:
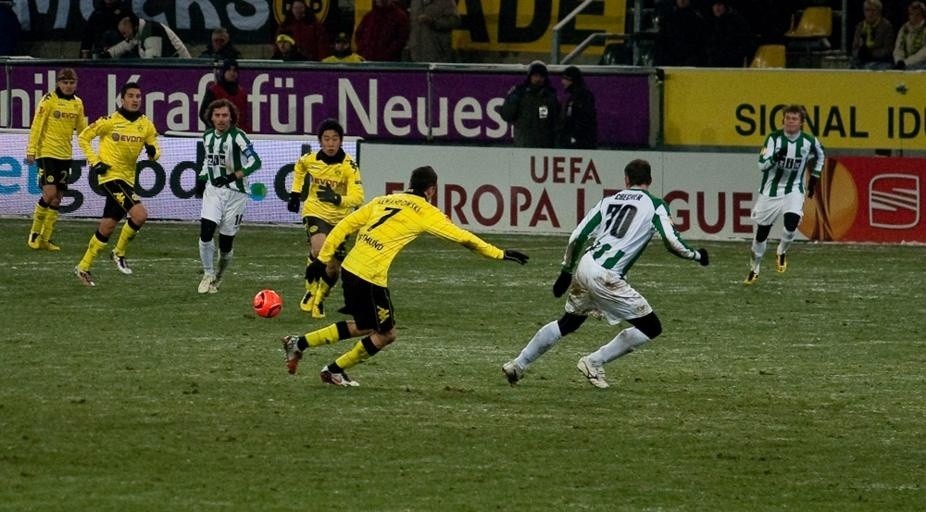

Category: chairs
[750, 44, 787, 69]
[784, 6, 832, 53]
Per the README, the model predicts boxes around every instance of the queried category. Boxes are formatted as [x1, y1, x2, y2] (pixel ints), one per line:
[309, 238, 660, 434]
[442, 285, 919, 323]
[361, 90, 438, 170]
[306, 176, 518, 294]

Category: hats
[277, 29, 295, 45]
[558, 66, 584, 84]
[526, 61, 549, 80]
[334, 32, 349, 44]
[221, 58, 240, 78]
[212, 27, 229, 44]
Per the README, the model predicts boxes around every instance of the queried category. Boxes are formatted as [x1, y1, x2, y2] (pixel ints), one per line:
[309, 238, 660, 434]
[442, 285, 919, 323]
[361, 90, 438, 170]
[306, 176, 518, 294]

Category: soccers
[254, 289, 283, 318]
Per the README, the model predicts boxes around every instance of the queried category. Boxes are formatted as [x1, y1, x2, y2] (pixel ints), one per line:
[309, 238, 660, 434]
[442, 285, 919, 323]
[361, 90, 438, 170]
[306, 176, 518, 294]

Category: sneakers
[208, 275, 223, 297]
[776, 251, 786, 273]
[197, 270, 217, 295]
[300, 290, 314, 312]
[743, 271, 759, 286]
[38, 241, 60, 252]
[576, 356, 610, 388]
[502, 361, 524, 388]
[110, 251, 132, 275]
[282, 335, 304, 376]
[312, 298, 326, 319]
[73, 265, 95, 287]
[320, 364, 360, 388]
[28, 230, 40, 249]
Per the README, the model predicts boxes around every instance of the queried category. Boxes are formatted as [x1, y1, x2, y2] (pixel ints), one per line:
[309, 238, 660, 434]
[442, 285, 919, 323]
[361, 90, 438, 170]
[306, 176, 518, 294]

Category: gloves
[772, 147, 788, 163]
[316, 182, 342, 206]
[143, 142, 156, 159]
[92, 162, 111, 176]
[553, 270, 572, 298]
[503, 250, 530, 266]
[194, 179, 206, 200]
[287, 191, 302, 214]
[696, 248, 710, 266]
[808, 176, 819, 200]
[211, 173, 238, 188]
[304, 258, 327, 284]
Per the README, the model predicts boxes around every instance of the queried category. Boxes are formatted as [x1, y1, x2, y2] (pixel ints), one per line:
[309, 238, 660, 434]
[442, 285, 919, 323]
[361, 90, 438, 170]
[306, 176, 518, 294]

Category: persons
[321, 30, 366, 63]
[288, 119, 364, 318]
[652, 0, 714, 74]
[197, 28, 240, 61]
[198, 58, 248, 136]
[74, 82, 161, 288]
[711, 1, 761, 68]
[744, 104, 825, 286]
[27, 66, 87, 252]
[845, 0, 894, 70]
[280, 165, 530, 388]
[103, 15, 195, 79]
[557, 66, 598, 149]
[354, 0, 410, 62]
[500, 60, 566, 147]
[195, 99, 262, 295]
[893, 1, 926, 71]
[499, 158, 710, 391]
[80, 0, 133, 60]
[408, 0, 461, 64]
[270, 31, 314, 61]
[278, 1, 328, 60]
